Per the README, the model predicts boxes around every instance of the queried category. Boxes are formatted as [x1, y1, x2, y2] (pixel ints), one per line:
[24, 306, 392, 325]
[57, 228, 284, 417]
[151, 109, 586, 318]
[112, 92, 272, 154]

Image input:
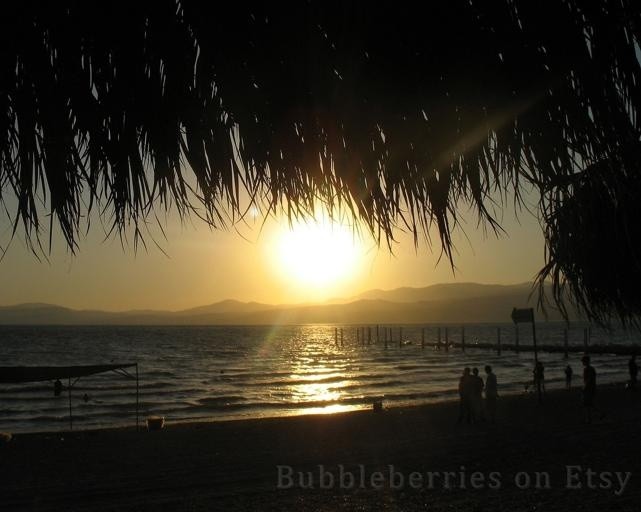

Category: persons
[626, 354, 640, 392]
[532, 362, 545, 400]
[484, 365, 500, 423]
[580, 355, 607, 426]
[458, 367, 474, 417]
[563, 364, 572, 392]
[81, 393, 89, 403]
[471, 367, 484, 413]
